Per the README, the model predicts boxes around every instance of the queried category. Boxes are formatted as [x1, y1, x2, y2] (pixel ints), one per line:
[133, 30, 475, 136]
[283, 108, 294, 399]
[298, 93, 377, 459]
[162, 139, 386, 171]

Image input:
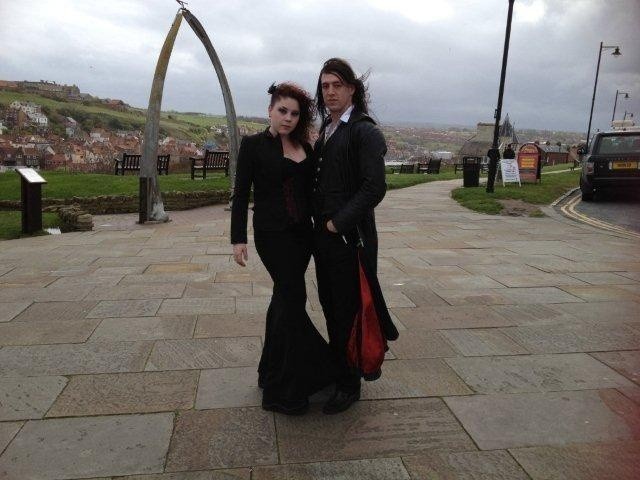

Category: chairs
[631, 139, 640, 150]
[602, 139, 614, 152]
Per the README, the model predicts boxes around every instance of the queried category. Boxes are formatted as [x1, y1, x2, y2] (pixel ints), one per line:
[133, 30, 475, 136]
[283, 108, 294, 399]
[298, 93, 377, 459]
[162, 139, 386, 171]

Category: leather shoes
[322, 390, 361, 415]
[262, 400, 308, 416]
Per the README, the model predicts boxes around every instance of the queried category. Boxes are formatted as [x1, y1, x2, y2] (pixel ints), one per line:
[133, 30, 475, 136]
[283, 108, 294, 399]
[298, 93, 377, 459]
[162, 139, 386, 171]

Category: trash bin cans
[463, 156, 481, 187]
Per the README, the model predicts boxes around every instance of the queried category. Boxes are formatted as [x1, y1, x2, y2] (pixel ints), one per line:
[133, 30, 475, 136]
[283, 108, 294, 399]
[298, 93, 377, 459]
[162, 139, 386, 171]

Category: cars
[577, 133, 639, 200]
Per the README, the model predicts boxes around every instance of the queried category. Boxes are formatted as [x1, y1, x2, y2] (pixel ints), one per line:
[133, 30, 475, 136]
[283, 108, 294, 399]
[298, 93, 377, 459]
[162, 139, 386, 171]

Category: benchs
[114, 153, 170, 176]
[189, 149, 229, 180]
[391, 157, 484, 174]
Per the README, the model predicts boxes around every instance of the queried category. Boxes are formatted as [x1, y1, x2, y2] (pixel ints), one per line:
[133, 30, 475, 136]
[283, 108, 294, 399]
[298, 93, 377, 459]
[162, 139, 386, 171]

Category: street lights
[584, 42, 621, 154]
[612, 91, 631, 124]
[624, 111, 633, 120]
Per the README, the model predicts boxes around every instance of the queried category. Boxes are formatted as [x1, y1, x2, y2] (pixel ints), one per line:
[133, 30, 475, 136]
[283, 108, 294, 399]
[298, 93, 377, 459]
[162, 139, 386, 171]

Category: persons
[230, 81, 356, 413]
[503, 143, 516, 159]
[487, 145, 501, 179]
[309, 58, 399, 381]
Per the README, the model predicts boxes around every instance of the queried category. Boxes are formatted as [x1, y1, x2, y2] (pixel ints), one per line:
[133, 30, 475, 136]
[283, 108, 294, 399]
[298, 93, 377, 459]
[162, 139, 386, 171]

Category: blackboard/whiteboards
[501, 159, 520, 183]
